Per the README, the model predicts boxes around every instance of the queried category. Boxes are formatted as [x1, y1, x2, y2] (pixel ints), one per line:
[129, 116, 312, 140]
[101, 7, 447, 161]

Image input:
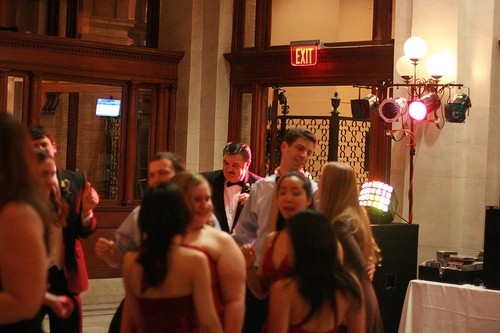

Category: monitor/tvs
[95, 97, 121, 118]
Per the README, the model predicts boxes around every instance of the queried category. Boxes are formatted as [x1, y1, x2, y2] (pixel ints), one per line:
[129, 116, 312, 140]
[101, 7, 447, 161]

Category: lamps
[351, 37, 472, 223]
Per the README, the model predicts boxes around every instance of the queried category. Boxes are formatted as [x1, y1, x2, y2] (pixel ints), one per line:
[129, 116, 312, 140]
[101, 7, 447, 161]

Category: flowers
[60, 179, 72, 197]
[246, 183, 254, 192]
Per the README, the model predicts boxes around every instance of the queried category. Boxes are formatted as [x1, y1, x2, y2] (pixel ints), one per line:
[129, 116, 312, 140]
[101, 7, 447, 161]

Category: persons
[0, 110, 383, 333]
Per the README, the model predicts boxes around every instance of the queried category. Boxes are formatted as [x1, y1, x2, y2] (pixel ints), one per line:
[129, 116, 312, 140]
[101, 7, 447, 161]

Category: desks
[397, 280, 500, 333]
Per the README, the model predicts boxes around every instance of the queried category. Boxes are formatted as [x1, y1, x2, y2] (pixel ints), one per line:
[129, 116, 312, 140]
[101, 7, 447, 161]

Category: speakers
[482, 206, 500, 291]
[370, 222, 419, 333]
